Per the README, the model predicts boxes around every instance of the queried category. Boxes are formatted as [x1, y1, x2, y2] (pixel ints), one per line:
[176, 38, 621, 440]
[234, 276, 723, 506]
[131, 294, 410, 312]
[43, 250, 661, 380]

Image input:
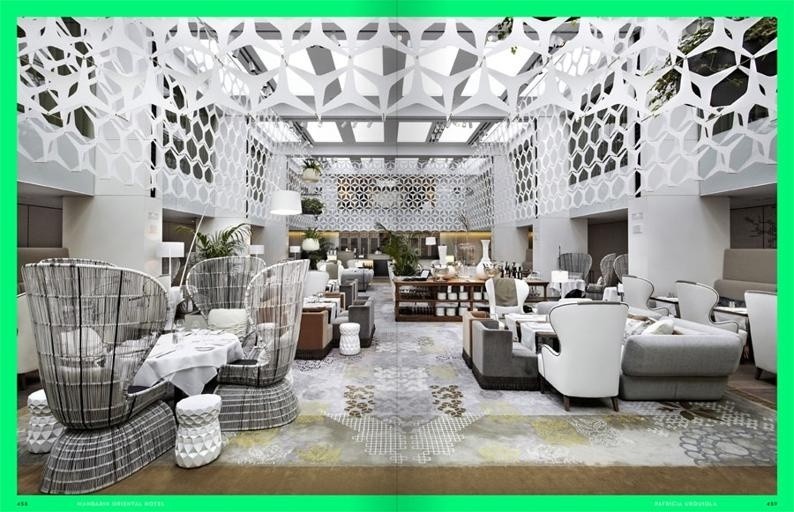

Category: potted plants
[301, 226, 320, 251]
[300, 158, 322, 182]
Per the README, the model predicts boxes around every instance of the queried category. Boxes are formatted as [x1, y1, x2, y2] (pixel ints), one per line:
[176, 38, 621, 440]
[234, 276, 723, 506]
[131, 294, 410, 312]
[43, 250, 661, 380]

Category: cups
[210, 323, 216, 331]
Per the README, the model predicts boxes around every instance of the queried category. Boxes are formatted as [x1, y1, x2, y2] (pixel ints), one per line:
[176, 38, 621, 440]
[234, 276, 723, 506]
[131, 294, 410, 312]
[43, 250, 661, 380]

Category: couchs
[713, 247, 777, 306]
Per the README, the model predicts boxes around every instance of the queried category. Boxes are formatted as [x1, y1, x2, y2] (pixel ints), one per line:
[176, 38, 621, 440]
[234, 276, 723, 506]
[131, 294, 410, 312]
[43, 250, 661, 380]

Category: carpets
[18, 281, 776, 467]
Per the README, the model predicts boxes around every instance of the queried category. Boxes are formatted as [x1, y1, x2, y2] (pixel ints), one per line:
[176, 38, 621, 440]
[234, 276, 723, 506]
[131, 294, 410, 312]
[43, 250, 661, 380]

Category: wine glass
[171, 319, 200, 343]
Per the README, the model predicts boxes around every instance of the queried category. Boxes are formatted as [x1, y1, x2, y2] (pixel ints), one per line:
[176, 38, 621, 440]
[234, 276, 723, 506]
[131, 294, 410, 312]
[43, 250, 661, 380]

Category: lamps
[239, 175, 302, 215]
[155, 241, 184, 321]
[426, 237, 436, 246]
[290, 246, 301, 261]
[248, 244, 264, 275]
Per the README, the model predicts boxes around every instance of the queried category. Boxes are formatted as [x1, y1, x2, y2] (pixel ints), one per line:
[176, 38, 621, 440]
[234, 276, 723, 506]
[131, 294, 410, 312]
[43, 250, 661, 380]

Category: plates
[195, 346, 214, 351]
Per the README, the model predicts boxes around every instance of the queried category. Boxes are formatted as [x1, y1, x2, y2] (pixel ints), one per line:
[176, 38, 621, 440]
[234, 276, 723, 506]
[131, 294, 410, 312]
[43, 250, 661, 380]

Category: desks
[389, 275, 549, 322]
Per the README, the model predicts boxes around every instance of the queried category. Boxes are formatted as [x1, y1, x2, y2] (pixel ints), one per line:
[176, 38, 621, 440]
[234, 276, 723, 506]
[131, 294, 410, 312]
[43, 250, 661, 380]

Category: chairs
[255, 259, 374, 360]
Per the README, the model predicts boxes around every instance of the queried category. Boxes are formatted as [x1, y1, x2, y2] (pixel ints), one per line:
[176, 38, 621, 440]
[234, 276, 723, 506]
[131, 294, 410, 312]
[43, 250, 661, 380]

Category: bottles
[499, 261, 522, 279]
[729, 299, 736, 308]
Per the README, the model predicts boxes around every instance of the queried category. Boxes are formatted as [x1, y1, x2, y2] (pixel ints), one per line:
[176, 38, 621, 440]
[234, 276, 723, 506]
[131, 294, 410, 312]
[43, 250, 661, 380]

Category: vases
[430, 245, 456, 279]
